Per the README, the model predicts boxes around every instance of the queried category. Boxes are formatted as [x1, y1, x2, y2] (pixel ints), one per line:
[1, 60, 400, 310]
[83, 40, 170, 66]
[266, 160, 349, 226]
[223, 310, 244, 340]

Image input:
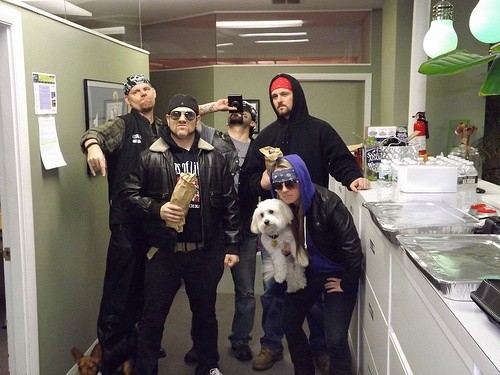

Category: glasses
[272, 179, 299, 190]
[167, 111, 197, 121]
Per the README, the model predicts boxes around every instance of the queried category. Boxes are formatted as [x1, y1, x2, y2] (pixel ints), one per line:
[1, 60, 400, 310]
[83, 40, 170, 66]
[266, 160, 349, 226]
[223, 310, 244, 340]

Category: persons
[115, 94, 239, 375]
[270, 154, 364, 375]
[80, 75, 166, 375]
[239, 74, 370, 375]
[184, 100, 257, 364]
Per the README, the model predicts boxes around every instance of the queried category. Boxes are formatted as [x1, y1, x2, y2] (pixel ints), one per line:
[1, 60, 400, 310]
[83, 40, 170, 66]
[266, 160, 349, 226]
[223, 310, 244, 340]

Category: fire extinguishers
[412, 111, 429, 156]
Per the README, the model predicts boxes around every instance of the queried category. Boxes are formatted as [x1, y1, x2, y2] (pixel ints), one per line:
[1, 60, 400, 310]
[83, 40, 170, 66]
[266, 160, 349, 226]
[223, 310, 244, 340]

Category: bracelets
[87, 142, 101, 149]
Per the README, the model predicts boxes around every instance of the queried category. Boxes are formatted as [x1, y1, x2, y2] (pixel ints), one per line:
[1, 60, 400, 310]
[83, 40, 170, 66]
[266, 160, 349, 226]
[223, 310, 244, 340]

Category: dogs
[70, 341, 133, 375]
[249, 197, 310, 295]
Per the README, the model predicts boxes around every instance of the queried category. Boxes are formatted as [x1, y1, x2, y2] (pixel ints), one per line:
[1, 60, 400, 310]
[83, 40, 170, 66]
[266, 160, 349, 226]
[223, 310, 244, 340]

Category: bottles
[448, 143, 482, 180]
[379, 155, 478, 213]
[227, 94, 244, 118]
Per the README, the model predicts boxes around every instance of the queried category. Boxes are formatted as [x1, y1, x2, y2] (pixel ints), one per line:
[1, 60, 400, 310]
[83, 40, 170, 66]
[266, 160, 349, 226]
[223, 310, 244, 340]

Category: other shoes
[315, 353, 329, 375]
[184, 346, 219, 365]
[158, 347, 166, 358]
[232, 344, 252, 361]
[208, 367, 223, 375]
[253, 348, 283, 370]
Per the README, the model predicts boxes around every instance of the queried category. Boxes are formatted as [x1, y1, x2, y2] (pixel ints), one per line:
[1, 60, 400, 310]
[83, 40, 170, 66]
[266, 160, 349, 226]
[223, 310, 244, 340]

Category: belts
[146, 242, 204, 261]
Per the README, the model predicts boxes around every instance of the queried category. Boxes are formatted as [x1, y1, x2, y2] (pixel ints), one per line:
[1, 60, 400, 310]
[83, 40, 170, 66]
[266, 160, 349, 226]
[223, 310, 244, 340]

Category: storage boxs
[398, 166, 458, 192]
[347, 126, 396, 179]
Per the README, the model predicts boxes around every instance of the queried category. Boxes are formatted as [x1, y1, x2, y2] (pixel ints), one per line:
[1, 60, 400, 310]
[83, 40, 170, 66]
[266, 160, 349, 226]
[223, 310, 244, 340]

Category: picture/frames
[84, 79, 133, 178]
[242, 99, 260, 133]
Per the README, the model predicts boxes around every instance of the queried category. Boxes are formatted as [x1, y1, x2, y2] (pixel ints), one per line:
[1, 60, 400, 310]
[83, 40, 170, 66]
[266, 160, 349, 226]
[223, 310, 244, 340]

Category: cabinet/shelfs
[334, 180, 482, 375]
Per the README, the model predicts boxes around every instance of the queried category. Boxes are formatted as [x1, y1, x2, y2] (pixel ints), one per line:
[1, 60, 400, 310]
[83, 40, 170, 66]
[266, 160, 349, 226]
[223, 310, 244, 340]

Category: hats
[168, 93, 198, 116]
[243, 100, 257, 121]
[124, 74, 151, 95]
[270, 76, 292, 93]
